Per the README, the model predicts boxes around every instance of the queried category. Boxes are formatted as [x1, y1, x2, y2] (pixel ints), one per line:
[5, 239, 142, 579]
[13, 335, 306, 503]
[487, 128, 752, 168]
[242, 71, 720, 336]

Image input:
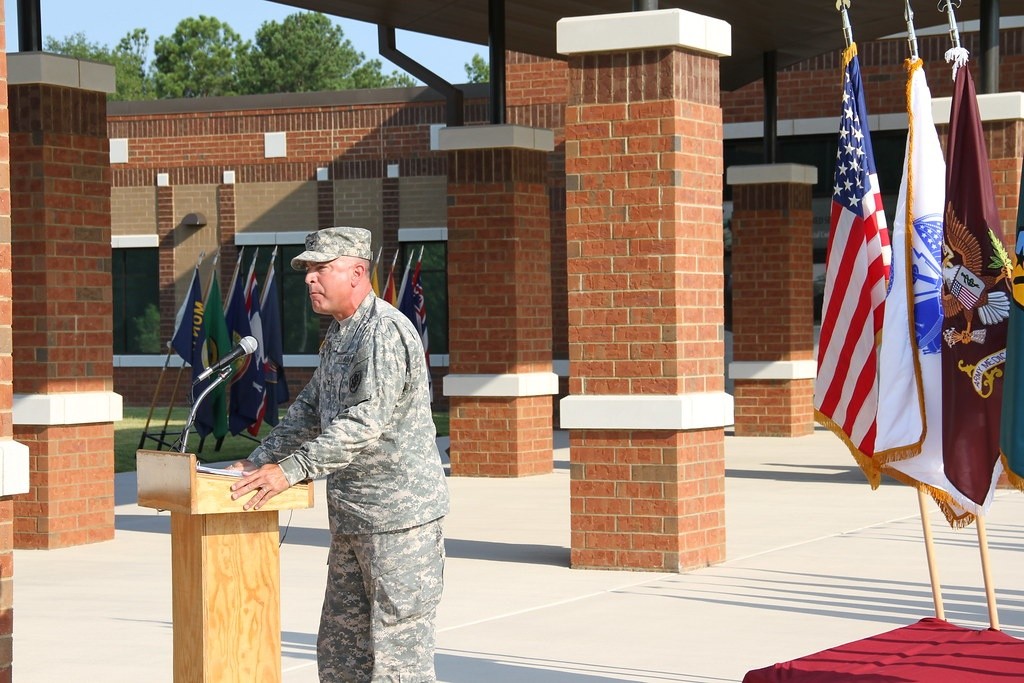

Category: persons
[225, 226, 450, 683]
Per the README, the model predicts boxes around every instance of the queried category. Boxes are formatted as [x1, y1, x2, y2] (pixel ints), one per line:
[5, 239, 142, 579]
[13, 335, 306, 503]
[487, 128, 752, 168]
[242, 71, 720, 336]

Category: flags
[941, 48, 1014, 507]
[813, 40, 892, 490]
[997, 160, 1024, 492]
[171, 262, 289, 439]
[368, 261, 434, 407]
[874, 59, 1004, 530]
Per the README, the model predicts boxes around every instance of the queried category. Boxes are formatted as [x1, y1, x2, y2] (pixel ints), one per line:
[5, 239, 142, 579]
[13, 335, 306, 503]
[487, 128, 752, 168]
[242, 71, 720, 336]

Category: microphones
[193, 336, 258, 385]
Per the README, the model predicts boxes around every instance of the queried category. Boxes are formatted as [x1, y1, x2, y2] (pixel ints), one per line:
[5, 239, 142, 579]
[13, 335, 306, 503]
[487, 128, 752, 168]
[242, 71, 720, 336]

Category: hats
[290, 227, 374, 271]
